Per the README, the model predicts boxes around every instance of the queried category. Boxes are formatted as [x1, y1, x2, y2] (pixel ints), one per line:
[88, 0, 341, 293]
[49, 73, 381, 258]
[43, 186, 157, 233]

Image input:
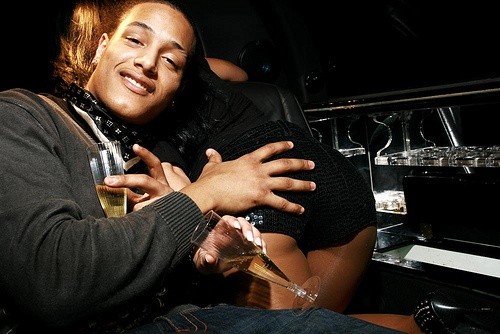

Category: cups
[87, 138, 128, 218]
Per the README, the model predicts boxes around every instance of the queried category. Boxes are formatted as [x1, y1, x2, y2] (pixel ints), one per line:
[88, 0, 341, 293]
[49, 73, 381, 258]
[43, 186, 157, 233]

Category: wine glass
[190, 210, 322, 318]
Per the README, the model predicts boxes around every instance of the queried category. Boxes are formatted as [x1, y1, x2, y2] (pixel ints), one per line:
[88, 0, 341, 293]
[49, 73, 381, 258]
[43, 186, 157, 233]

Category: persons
[0, 1, 404, 333]
[56, 1, 500, 333]
